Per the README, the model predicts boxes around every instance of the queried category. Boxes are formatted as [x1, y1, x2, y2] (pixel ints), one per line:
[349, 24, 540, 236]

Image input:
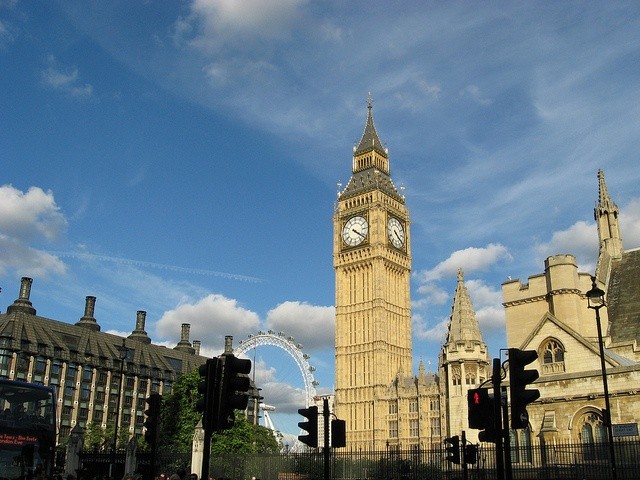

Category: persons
[32, 469, 113, 480]
[124, 472, 142, 480]
[11, 405, 28, 421]
[28, 407, 48, 424]
[0, 408, 11, 420]
[158, 470, 218, 480]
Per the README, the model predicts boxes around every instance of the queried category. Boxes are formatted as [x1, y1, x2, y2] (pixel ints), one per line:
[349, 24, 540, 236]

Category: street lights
[110, 343, 129, 480]
[585, 275, 617, 480]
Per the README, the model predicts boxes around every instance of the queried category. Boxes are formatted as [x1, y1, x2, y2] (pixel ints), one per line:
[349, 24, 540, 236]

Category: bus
[0, 374, 60, 480]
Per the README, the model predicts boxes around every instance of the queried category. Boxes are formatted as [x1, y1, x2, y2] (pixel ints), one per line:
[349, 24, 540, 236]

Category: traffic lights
[298, 406, 318, 448]
[215, 354, 252, 430]
[144, 392, 160, 444]
[478, 425, 495, 442]
[196, 358, 211, 429]
[445, 435, 460, 464]
[509, 348, 540, 429]
[468, 389, 490, 430]
